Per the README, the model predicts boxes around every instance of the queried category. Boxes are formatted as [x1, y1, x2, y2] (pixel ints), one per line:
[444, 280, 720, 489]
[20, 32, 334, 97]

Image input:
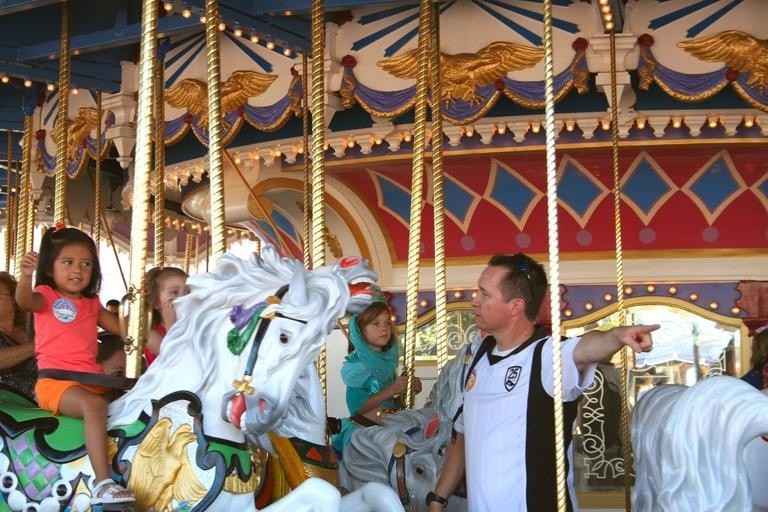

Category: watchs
[426, 492, 449, 507]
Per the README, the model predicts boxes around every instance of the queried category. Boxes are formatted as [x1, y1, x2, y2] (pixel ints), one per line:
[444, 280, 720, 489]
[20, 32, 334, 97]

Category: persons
[330, 301, 422, 463]
[16, 220, 140, 505]
[144, 267, 191, 371]
[1, 272, 39, 405]
[96, 331, 146, 402]
[107, 301, 121, 317]
[426, 252, 661, 512]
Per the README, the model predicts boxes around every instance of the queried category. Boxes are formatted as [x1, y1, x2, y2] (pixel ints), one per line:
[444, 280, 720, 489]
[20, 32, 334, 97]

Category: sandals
[92, 478, 138, 505]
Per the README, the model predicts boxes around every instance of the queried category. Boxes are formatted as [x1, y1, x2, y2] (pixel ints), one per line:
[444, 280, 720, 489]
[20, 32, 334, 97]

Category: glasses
[515, 254, 538, 310]
